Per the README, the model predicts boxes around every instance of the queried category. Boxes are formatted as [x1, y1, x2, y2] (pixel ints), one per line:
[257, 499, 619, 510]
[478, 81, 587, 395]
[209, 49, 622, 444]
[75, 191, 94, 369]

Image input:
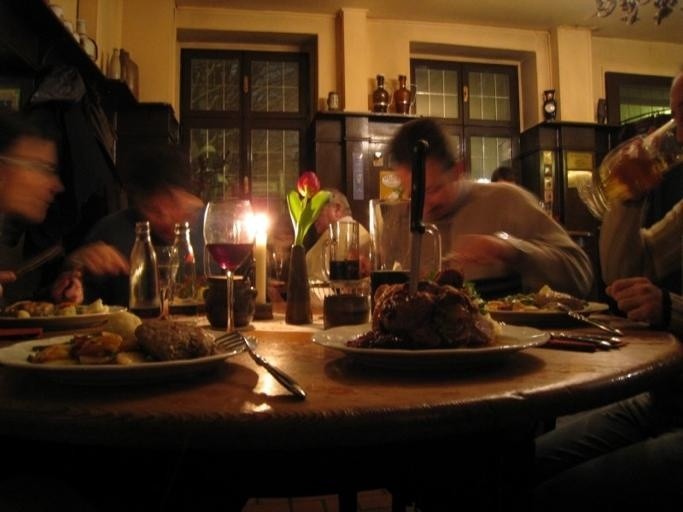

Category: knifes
[0, 319, 110, 339]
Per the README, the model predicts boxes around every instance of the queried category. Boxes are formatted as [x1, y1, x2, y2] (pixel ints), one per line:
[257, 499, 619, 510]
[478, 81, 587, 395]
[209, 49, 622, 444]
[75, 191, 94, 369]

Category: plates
[0, 333, 257, 372]
[1, 306, 127, 327]
[482, 299, 612, 325]
[313, 320, 550, 361]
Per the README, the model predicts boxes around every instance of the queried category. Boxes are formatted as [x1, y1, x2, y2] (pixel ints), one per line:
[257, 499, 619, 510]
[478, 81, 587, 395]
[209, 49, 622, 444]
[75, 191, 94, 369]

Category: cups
[575, 120, 681, 218]
[50, 5, 79, 44]
[315, 219, 373, 324]
[368, 199, 442, 315]
[327, 91, 339, 111]
[204, 274, 255, 328]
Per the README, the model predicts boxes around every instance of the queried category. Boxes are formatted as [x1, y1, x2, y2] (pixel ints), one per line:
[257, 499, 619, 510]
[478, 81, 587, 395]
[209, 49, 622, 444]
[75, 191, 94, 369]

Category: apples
[93, 312, 140, 352]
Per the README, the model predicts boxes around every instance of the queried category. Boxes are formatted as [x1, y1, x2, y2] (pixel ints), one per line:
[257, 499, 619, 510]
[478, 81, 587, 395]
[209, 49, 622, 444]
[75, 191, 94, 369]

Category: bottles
[372, 74, 389, 114]
[394, 73, 414, 115]
[173, 222, 197, 299]
[78, 18, 121, 81]
[127, 221, 160, 319]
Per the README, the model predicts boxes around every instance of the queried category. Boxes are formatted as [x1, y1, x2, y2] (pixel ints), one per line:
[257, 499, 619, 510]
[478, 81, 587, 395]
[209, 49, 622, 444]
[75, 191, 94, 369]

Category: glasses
[1, 152, 55, 179]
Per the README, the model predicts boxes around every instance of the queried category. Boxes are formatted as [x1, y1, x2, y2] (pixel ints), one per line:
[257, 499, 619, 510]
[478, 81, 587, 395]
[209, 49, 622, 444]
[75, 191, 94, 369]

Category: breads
[135, 321, 218, 359]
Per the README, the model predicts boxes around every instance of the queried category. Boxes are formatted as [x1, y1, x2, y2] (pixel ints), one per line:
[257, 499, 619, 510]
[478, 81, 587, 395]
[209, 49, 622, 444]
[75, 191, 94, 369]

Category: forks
[213, 330, 306, 399]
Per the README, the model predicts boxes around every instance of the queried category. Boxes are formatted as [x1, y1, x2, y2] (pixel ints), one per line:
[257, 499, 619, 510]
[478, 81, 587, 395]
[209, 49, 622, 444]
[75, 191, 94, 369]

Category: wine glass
[154, 247, 180, 319]
[203, 201, 257, 352]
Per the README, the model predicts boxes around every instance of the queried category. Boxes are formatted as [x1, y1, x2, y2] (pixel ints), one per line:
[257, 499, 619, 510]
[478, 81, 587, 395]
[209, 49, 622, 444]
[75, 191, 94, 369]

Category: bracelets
[650, 288, 672, 333]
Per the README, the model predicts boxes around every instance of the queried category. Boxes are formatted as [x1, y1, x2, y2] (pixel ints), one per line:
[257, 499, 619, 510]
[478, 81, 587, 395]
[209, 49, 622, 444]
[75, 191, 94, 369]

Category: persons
[496, 72, 683, 512]
[382, 118, 595, 302]
[304, 190, 379, 284]
[490, 165, 519, 187]
[0, 116, 84, 313]
[65, 172, 206, 309]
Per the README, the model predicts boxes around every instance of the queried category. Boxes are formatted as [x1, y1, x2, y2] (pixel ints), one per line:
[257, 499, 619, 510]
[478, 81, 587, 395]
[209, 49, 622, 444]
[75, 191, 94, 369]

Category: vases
[372, 74, 394, 113]
[326, 91, 340, 112]
[42, 1, 139, 102]
[285, 244, 313, 325]
[392, 74, 419, 115]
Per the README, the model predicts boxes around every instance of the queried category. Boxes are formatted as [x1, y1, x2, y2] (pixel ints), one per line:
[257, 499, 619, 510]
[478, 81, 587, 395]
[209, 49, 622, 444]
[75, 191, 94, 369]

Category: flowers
[283, 169, 333, 248]
